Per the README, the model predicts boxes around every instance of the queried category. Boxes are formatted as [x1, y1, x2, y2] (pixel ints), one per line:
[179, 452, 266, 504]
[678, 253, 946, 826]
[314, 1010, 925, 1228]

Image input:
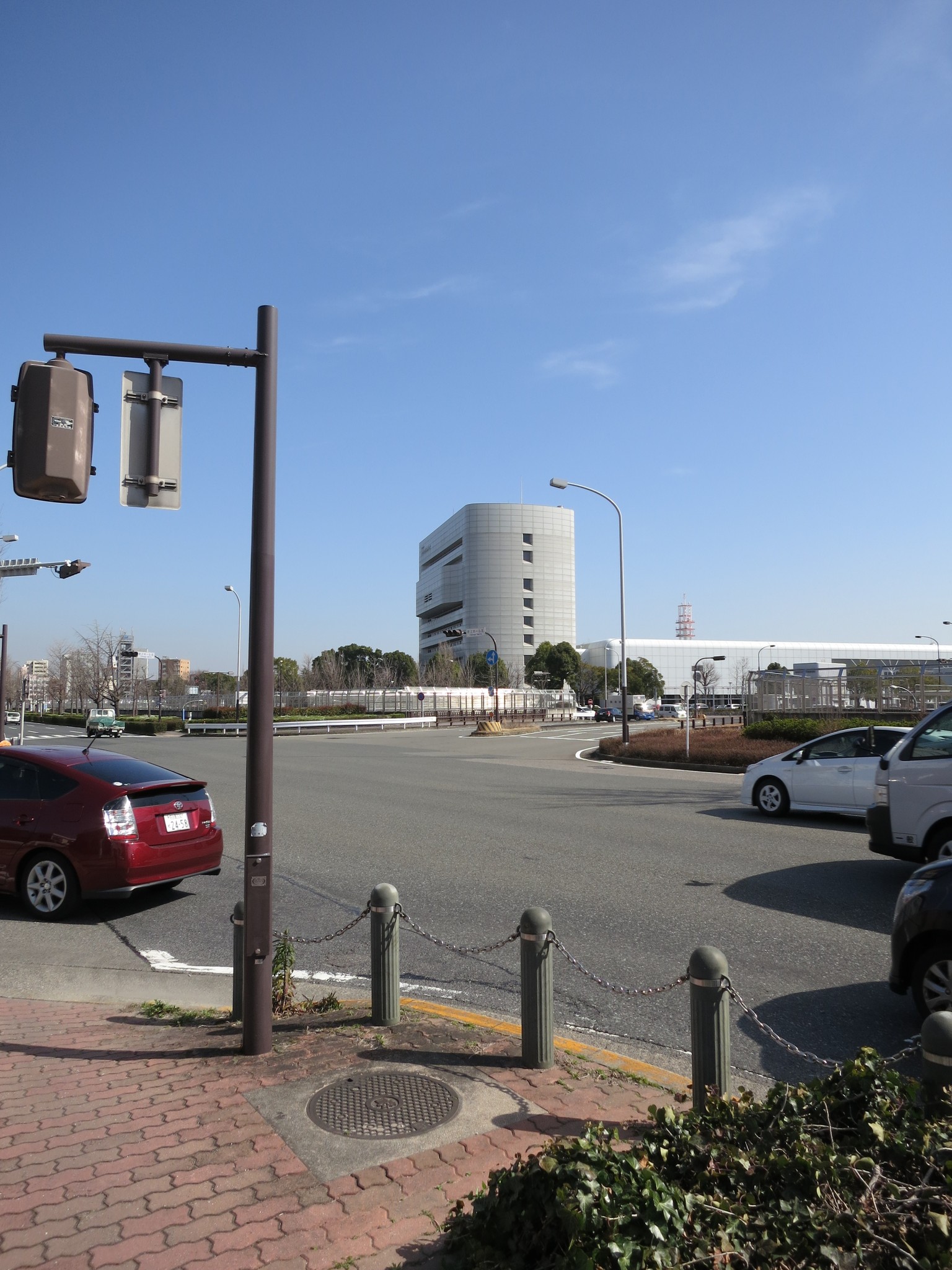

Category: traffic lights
[442, 629, 462, 637]
[122, 650, 138, 657]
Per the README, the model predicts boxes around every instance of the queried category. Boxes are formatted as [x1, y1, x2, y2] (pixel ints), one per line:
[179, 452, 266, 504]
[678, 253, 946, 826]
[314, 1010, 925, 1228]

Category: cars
[594, 707, 623, 723]
[738, 725, 951, 826]
[889, 851, 952, 1036]
[572, 706, 596, 720]
[4, 711, 21, 725]
[652, 702, 746, 716]
[0, 738, 224, 920]
[842, 704, 855, 709]
[584, 705, 601, 709]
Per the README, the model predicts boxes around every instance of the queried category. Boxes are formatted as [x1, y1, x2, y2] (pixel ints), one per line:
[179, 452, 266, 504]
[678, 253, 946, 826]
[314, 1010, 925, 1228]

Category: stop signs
[588, 699, 593, 705]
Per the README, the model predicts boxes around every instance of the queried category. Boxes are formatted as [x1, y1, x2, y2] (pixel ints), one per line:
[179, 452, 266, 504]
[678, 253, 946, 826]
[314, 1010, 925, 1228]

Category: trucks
[605, 694, 655, 721]
[86, 708, 125, 739]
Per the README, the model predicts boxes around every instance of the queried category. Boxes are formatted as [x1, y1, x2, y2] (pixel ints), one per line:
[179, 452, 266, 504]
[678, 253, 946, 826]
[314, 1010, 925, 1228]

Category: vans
[658, 704, 687, 719]
[864, 697, 952, 864]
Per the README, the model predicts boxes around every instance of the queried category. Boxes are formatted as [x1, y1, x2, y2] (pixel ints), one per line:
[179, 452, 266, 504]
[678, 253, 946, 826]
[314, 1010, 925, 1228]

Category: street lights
[224, 585, 241, 736]
[758, 645, 775, 671]
[693, 655, 726, 719]
[604, 647, 621, 696]
[728, 681, 733, 709]
[551, 476, 630, 750]
[202, 670, 235, 718]
[605, 637, 627, 709]
[915, 635, 941, 685]
